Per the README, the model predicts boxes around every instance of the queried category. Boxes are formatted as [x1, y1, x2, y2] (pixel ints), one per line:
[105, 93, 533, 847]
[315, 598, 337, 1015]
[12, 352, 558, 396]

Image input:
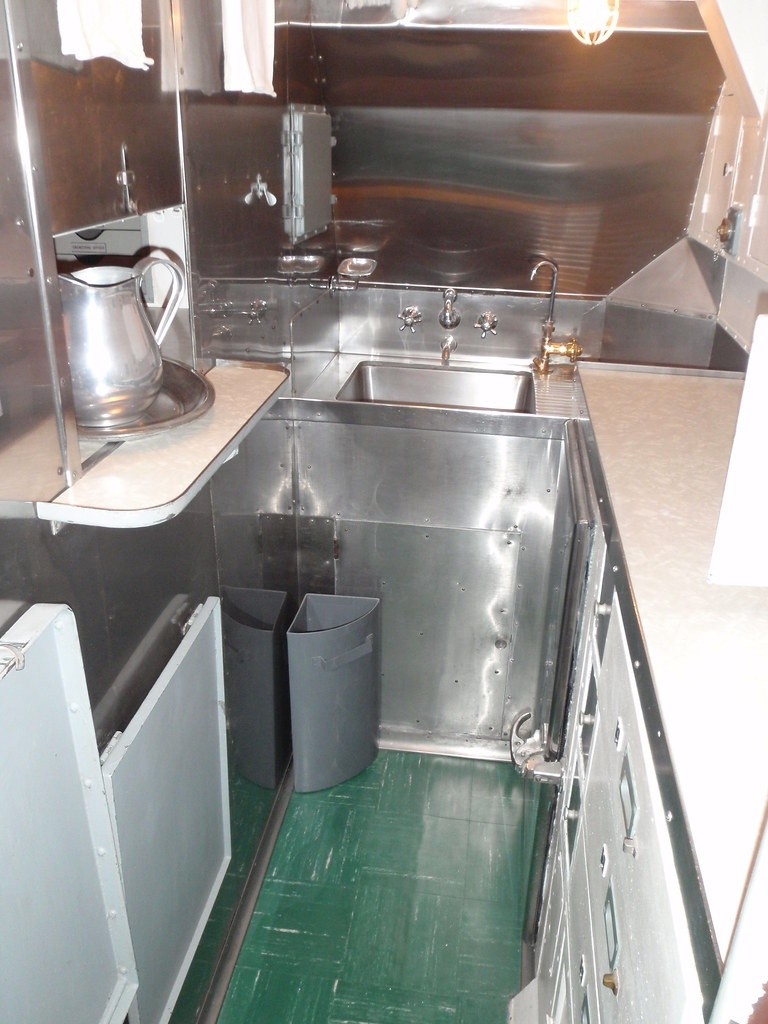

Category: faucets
[527, 251, 558, 270]
[529, 261, 583, 373]
[441, 335, 457, 361]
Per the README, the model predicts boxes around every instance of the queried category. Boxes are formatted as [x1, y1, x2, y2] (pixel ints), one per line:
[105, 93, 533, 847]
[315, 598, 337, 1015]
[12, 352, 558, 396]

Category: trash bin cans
[286, 592, 380, 794]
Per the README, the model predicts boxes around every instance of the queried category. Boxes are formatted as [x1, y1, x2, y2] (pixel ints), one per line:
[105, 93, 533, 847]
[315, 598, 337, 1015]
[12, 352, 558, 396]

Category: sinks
[334, 360, 537, 415]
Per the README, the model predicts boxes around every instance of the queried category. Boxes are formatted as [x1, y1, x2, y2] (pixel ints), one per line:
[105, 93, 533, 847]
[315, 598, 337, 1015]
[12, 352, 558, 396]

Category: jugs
[56, 258, 185, 426]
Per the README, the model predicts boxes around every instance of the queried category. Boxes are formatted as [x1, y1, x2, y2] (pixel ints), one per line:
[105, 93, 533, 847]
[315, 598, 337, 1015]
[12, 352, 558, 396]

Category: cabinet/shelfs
[688, 80, 767, 280]
[533, 526, 705, 1023]
